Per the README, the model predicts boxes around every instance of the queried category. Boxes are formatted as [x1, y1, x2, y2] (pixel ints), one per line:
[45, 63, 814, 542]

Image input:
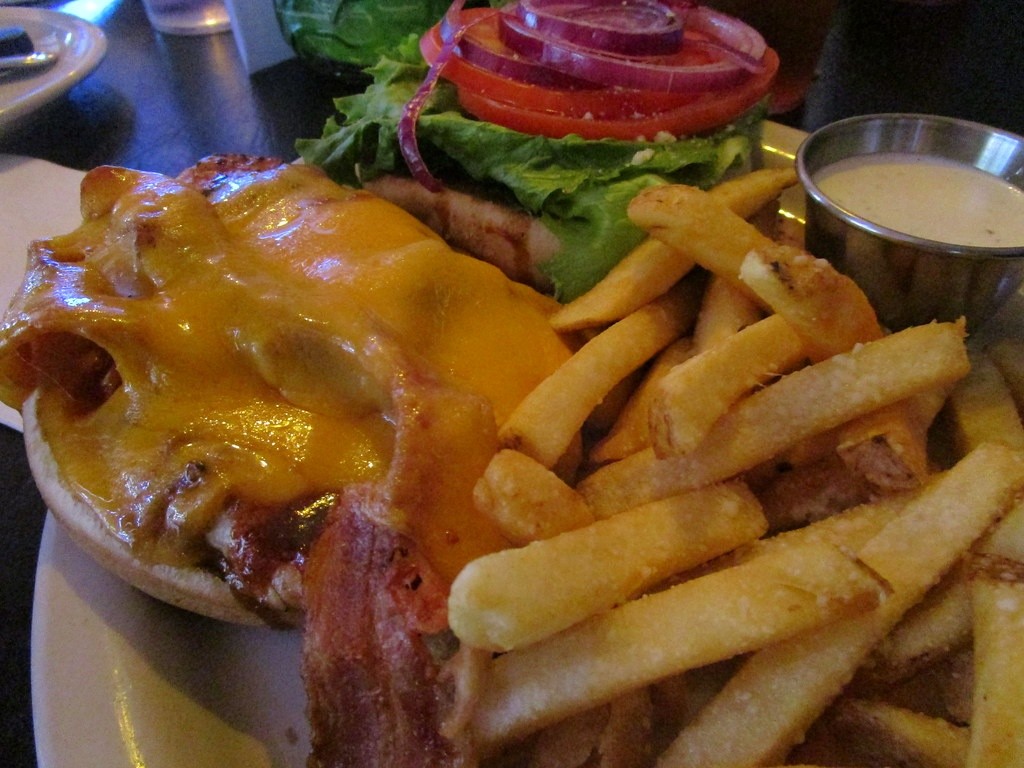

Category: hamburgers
[273, 0, 770, 302]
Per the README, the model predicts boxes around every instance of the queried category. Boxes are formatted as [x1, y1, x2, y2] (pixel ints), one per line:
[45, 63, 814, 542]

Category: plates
[0, 6, 109, 138]
[30, 505, 311, 768]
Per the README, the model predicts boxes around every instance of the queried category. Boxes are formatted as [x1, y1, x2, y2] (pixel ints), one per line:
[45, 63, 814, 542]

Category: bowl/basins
[272, 0, 493, 82]
[793, 112, 1024, 344]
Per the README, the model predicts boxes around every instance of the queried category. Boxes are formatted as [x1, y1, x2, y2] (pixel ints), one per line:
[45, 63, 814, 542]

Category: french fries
[440, 162, 1024, 768]
